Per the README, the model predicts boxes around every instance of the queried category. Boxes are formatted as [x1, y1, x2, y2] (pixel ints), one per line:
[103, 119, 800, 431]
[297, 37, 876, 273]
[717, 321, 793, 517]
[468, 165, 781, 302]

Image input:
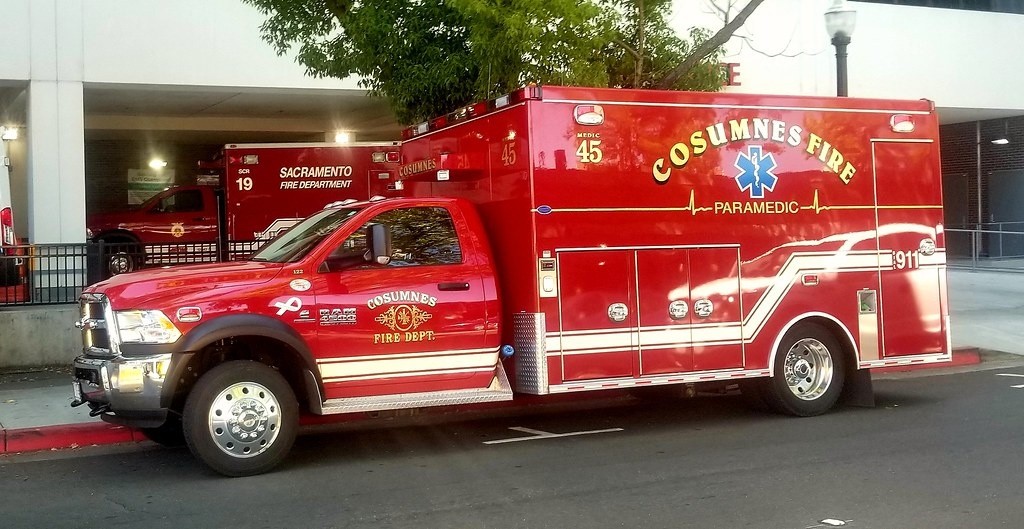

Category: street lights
[826, 0, 859, 98]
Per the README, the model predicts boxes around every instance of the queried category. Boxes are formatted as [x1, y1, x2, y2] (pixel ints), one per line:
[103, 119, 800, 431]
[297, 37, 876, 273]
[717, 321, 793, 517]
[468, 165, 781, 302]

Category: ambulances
[72, 83, 954, 476]
[87, 140, 403, 280]
